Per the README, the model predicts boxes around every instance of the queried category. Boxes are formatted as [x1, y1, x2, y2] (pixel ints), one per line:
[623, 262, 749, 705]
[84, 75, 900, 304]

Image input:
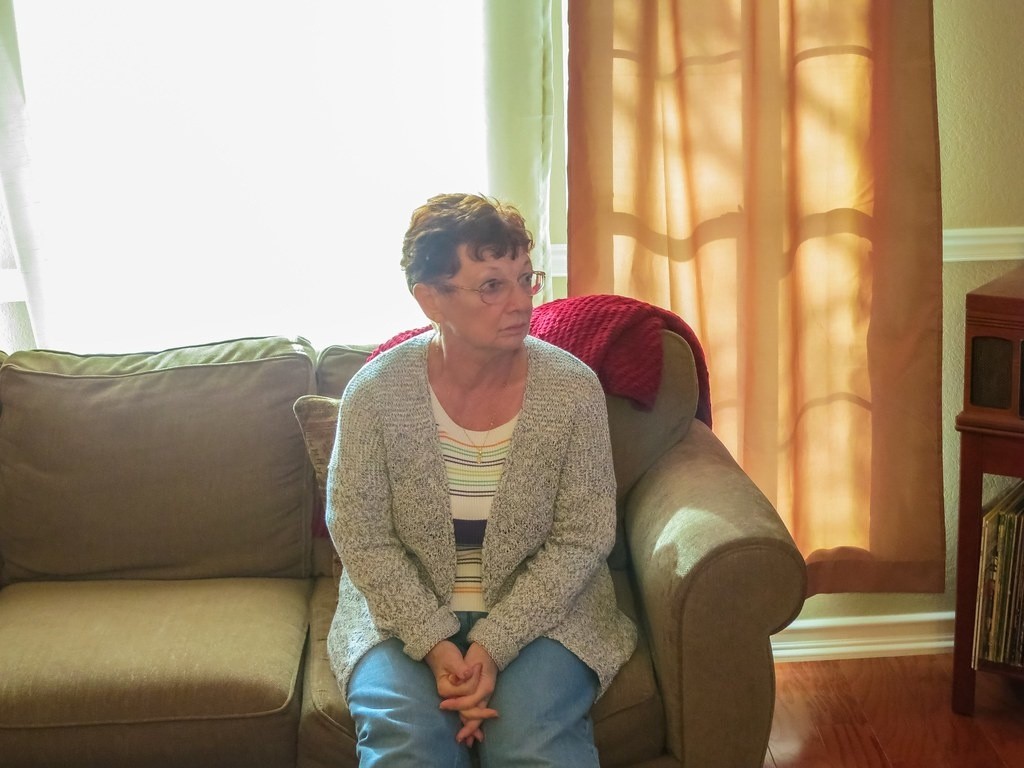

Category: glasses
[438, 269, 546, 305]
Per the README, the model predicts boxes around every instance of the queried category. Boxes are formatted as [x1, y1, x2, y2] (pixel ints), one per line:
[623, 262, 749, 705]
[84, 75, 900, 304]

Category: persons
[322, 192, 637, 768]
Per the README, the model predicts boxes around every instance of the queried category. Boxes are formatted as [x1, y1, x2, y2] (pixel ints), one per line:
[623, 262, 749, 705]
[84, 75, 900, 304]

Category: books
[971, 480, 1024, 669]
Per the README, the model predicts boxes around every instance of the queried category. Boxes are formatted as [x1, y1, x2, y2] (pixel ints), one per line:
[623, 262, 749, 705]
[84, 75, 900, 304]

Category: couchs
[0, 294, 809, 768]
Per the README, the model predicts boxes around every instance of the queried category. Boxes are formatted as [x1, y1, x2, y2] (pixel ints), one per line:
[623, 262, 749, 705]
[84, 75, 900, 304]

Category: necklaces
[434, 344, 510, 464]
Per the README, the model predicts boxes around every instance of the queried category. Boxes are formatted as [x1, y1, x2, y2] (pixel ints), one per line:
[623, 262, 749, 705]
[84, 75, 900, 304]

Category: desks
[951, 260, 1024, 720]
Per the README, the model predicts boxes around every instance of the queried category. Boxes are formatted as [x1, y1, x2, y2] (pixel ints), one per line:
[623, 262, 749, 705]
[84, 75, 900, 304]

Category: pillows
[292, 394, 343, 588]
